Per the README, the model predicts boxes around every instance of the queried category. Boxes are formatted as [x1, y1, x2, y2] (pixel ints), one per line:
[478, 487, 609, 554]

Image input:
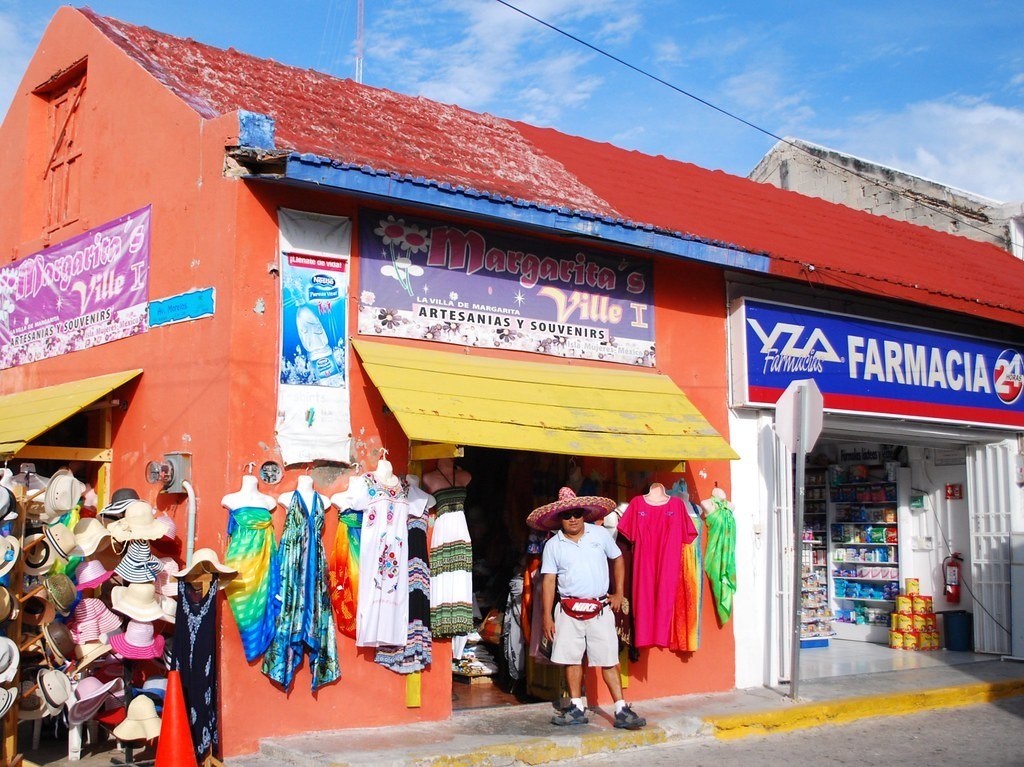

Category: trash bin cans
[935, 610, 973, 651]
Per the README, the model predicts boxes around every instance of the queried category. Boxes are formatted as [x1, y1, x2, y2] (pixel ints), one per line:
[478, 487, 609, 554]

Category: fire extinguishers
[942, 552, 964, 605]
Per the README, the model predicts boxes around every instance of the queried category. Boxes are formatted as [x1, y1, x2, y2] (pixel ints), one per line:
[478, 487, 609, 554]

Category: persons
[524, 487, 647, 729]
[262, 474, 340, 697]
[221, 475, 281, 664]
[423, 457, 475, 639]
[329, 461, 436, 673]
[668, 489, 703, 651]
[559, 466, 641, 660]
[700, 487, 737, 626]
[618, 482, 700, 648]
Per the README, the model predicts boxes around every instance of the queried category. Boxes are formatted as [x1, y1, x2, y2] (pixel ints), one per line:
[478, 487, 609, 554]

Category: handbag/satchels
[559, 598, 603, 620]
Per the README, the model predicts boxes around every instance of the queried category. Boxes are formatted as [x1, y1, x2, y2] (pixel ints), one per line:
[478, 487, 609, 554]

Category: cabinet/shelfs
[829, 466, 914, 641]
[0, 483, 55, 767]
[798, 472, 830, 648]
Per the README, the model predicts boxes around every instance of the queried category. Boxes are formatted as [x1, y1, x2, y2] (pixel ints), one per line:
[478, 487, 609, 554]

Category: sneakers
[614, 704, 646, 728]
[551, 703, 588, 725]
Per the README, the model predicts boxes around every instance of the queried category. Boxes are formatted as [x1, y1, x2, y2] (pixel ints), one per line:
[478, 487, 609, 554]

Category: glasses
[561, 509, 583, 520]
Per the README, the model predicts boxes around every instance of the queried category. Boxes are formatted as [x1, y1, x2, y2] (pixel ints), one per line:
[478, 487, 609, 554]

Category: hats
[0, 468, 237, 741]
[527, 486, 617, 532]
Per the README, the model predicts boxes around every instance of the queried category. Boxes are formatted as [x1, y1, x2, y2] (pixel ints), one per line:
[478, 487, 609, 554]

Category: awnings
[0, 369, 144, 462]
[352, 338, 740, 460]
